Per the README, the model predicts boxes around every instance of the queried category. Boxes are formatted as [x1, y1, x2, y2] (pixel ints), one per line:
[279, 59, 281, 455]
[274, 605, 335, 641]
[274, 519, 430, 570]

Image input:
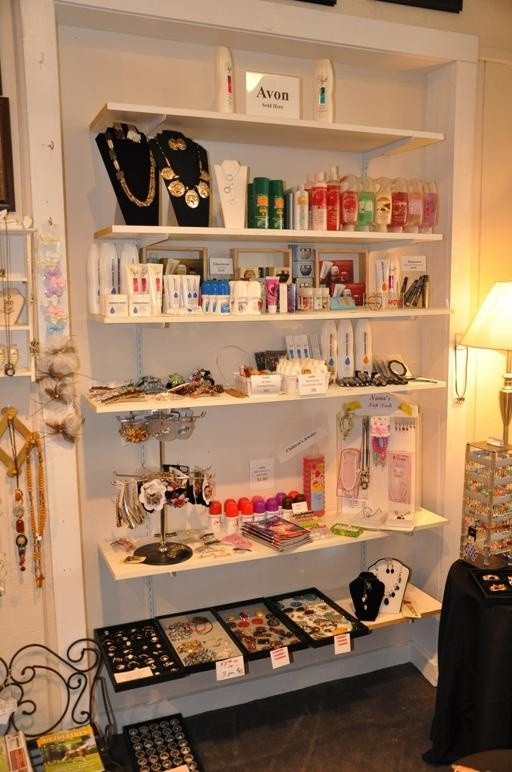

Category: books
[241, 514, 312, 553]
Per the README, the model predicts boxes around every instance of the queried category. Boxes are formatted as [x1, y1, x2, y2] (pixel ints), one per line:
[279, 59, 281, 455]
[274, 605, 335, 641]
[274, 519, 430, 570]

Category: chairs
[1, 638, 119, 772]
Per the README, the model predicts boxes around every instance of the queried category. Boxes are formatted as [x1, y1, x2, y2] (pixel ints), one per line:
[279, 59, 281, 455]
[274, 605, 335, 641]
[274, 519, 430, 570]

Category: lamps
[459, 281, 512, 445]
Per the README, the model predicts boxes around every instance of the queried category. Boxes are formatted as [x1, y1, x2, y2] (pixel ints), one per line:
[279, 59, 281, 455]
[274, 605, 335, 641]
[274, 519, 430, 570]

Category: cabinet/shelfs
[0, 1, 477, 733]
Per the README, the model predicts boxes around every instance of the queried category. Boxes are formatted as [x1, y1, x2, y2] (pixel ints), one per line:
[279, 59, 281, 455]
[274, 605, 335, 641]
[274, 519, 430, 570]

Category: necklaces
[363, 332, 369, 363]
[24, 432, 45, 588]
[156, 141, 211, 209]
[219, 164, 241, 205]
[357, 575, 378, 611]
[329, 334, 334, 366]
[7, 412, 28, 572]
[1, 216, 15, 377]
[344, 333, 350, 365]
[375, 563, 405, 604]
[454, 332, 468, 404]
[107, 136, 156, 207]
